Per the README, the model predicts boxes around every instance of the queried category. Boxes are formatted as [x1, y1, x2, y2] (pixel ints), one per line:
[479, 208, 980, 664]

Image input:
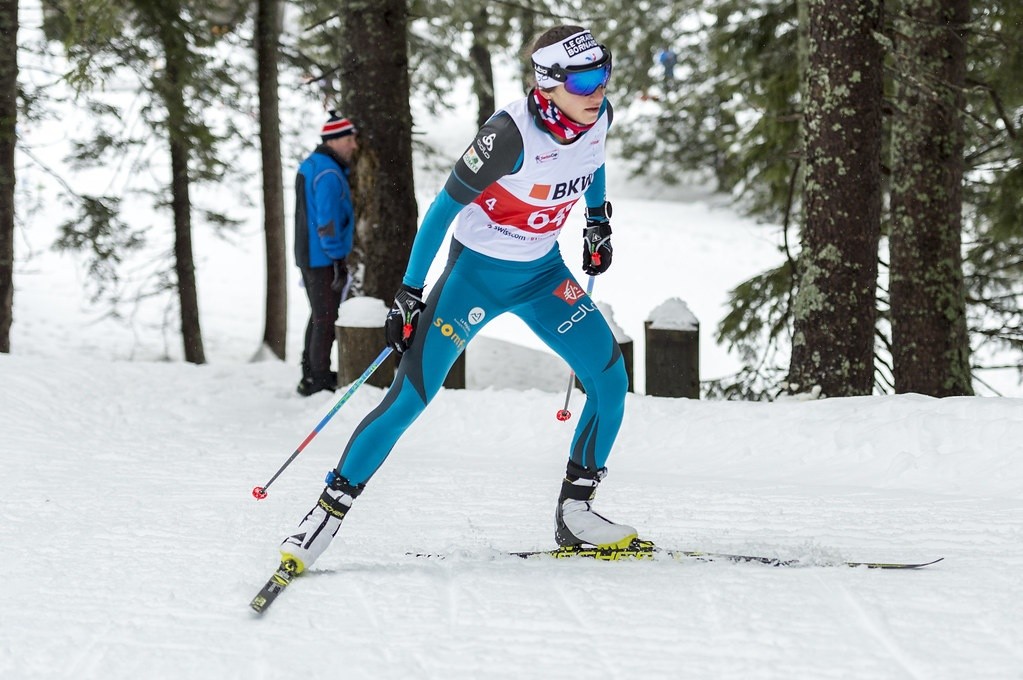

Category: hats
[321, 111, 355, 140]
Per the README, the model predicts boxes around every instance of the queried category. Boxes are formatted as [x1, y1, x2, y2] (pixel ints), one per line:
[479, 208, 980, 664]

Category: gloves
[331, 260, 347, 292]
[385, 284, 427, 355]
[582, 218, 613, 277]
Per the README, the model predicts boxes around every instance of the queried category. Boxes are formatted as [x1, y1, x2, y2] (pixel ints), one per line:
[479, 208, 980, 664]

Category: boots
[280, 469, 364, 574]
[556, 460, 638, 551]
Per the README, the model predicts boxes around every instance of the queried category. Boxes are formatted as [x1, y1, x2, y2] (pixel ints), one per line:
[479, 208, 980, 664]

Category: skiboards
[247, 547, 945, 615]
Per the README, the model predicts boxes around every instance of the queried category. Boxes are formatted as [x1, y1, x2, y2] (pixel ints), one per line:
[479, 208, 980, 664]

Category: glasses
[564, 49, 612, 96]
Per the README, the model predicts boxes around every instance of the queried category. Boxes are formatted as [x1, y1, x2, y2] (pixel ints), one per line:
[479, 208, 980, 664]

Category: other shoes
[297, 370, 339, 395]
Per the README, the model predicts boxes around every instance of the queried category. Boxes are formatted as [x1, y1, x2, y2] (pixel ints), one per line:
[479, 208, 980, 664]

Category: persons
[279, 26, 638, 575]
[294, 111, 358, 396]
